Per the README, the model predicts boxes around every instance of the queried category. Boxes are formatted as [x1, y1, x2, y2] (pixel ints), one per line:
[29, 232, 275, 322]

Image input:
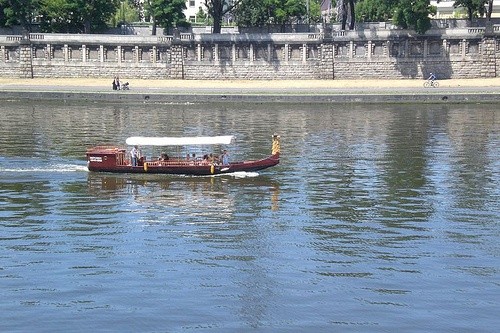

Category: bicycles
[423, 80, 440, 87]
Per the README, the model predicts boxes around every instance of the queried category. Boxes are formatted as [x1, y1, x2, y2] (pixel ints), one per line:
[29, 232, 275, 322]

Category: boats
[86, 135, 281, 175]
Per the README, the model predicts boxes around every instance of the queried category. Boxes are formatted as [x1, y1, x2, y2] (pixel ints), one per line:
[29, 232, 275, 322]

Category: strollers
[119, 80, 130, 90]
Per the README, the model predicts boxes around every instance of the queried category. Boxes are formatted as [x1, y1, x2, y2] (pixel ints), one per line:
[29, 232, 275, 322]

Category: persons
[112, 75, 129, 91]
[130, 145, 230, 166]
[427, 72, 436, 86]
